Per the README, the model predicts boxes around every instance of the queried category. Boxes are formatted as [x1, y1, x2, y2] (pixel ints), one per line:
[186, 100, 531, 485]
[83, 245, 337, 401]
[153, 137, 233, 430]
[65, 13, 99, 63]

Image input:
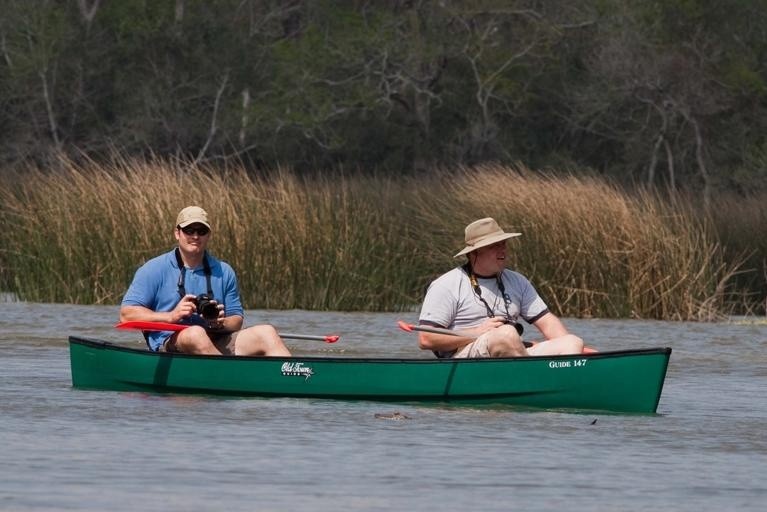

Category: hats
[176, 206, 210, 229]
[455, 218, 522, 258]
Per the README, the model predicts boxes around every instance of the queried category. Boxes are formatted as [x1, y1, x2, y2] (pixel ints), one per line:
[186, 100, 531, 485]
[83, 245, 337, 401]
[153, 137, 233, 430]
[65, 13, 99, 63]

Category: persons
[418, 217, 584, 358]
[119, 206, 291, 356]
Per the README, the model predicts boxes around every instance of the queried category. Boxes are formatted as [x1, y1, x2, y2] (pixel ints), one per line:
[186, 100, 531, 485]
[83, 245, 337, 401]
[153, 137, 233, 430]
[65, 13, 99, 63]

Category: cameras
[192, 293, 218, 320]
[502, 320, 523, 336]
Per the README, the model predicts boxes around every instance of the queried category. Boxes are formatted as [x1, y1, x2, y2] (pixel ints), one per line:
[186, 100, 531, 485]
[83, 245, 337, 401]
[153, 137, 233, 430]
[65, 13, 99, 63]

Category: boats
[67, 333, 671, 417]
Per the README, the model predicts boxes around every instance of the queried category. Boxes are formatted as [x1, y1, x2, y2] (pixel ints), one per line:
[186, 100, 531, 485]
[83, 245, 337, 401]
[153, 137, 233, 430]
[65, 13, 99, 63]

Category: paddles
[115, 321, 339, 343]
[397, 322, 599, 353]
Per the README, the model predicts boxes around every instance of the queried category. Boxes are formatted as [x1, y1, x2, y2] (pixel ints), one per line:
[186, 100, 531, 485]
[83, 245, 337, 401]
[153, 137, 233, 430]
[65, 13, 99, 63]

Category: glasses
[181, 225, 207, 235]
